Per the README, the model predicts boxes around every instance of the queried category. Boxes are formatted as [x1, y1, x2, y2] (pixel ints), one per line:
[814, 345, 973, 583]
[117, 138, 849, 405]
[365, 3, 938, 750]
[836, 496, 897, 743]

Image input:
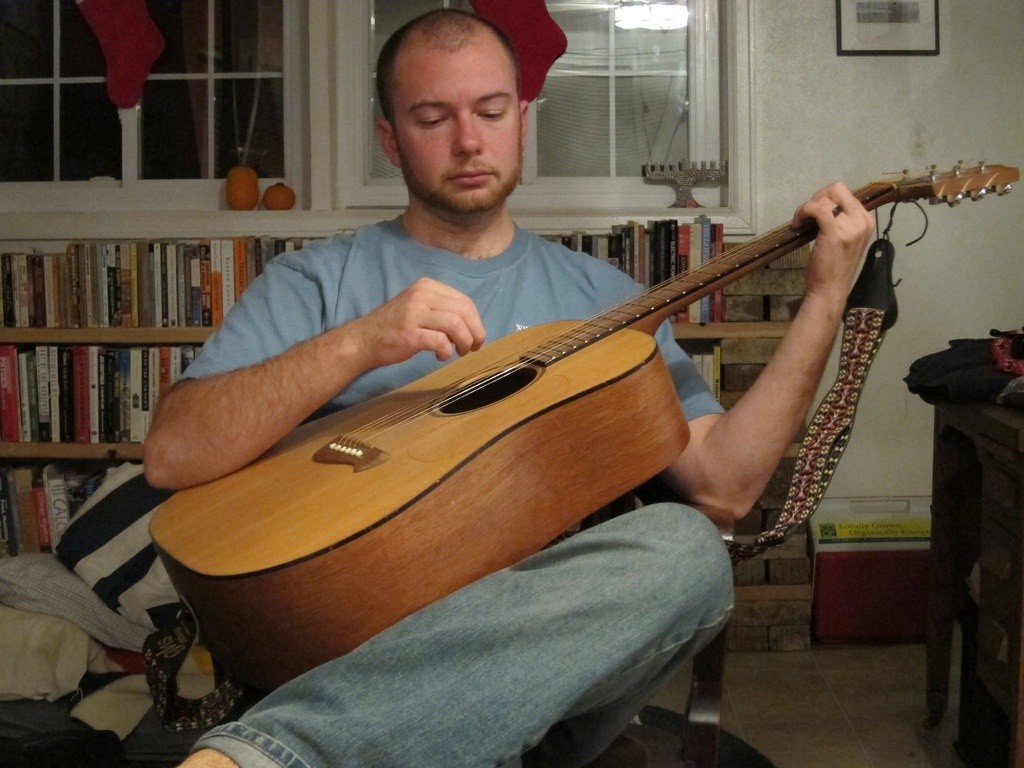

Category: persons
[144, 8, 878, 768]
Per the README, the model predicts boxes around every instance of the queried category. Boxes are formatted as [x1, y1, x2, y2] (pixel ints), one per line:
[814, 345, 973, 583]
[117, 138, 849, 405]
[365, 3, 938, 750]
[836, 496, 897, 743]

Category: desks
[924, 390, 1024, 768]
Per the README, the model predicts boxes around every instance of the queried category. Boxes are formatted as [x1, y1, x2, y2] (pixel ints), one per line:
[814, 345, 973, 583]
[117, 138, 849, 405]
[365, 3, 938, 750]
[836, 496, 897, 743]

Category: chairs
[584, 507, 740, 768]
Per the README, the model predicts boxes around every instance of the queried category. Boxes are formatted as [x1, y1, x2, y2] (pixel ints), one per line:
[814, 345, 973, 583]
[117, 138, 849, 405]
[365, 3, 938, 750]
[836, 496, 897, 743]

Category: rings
[832, 206, 843, 217]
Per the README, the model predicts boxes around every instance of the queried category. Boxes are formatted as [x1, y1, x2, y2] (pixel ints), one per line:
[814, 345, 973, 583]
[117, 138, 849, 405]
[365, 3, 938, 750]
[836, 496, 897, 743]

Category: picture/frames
[836, 0, 941, 57]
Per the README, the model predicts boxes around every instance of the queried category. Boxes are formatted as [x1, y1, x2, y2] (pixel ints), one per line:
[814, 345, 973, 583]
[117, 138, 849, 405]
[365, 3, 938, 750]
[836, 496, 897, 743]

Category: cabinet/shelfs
[0, 318, 811, 607]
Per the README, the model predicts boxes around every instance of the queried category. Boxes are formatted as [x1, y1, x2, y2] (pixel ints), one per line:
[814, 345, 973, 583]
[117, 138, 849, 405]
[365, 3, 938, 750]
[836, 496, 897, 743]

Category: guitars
[146, 164, 1024, 685]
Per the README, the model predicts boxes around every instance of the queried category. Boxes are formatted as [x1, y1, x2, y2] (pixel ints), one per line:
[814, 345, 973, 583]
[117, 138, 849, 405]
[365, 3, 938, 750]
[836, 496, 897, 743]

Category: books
[537, 215, 723, 405]
[1, 233, 327, 558]
